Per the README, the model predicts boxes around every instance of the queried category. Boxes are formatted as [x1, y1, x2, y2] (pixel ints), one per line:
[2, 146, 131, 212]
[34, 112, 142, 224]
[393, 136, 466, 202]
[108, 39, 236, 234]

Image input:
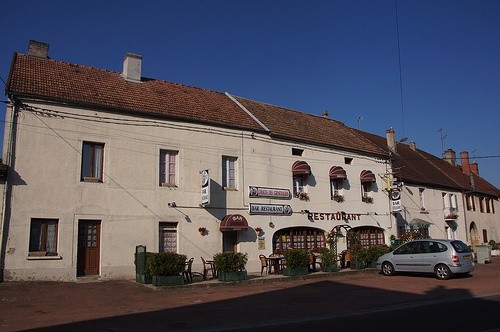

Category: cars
[376, 238, 476, 280]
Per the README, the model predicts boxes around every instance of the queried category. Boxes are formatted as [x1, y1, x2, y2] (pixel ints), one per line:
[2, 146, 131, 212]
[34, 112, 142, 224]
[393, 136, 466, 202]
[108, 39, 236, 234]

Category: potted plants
[319, 251, 337, 272]
[213, 252, 248, 281]
[134, 251, 187, 286]
[351, 244, 393, 269]
[282, 248, 310, 276]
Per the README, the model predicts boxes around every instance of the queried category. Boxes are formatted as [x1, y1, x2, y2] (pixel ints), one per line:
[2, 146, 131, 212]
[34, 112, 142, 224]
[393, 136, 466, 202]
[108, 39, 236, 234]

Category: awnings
[361, 170, 376, 185]
[292, 161, 311, 175]
[220, 214, 248, 232]
[330, 166, 348, 181]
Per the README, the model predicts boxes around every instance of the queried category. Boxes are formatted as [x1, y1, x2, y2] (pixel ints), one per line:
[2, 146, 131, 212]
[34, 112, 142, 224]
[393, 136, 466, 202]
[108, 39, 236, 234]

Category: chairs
[201, 257, 217, 280]
[182, 258, 194, 283]
[259, 254, 279, 275]
